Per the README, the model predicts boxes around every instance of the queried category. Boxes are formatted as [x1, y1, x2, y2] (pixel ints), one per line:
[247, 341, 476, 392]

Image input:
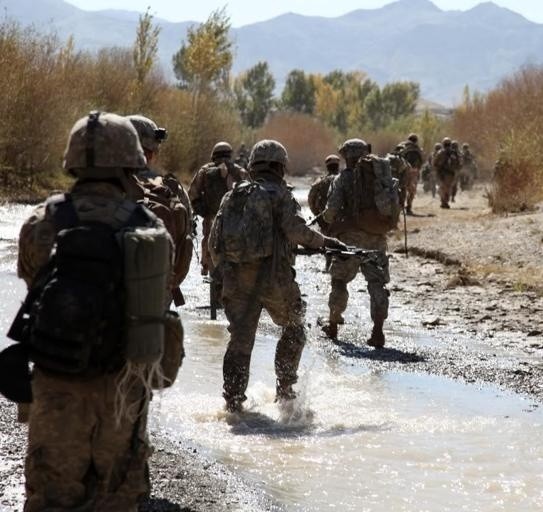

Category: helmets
[210, 142, 233, 159]
[341, 138, 370, 159]
[408, 133, 469, 148]
[62, 110, 162, 176]
[248, 139, 288, 169]
[325, 155, 340, 166]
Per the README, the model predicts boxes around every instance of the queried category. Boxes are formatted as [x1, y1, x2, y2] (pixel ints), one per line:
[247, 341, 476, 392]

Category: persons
[127, 115, 198, 307]
[395, 132, 474, 216]
[17, 111, 177, 511]
[307, 139, 401, 347]
[188, 140, 250, 309]
[208, 138, 346, 412]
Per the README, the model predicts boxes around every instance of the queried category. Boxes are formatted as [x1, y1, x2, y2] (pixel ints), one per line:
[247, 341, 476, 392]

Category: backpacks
[353, 153, 398, 231]
[22, 193, 157, 379]
[205, 166, 274, 266]
[319, 176, 334, 210]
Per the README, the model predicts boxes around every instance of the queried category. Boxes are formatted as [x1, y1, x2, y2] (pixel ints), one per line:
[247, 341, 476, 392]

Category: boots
[223, 386, 296, 412]
[322, 323, 385, 346]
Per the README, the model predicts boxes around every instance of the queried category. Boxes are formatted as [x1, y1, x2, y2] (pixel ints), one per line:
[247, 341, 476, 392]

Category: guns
[293, 247, 367, 269]
[210, 269, 219, 319]
